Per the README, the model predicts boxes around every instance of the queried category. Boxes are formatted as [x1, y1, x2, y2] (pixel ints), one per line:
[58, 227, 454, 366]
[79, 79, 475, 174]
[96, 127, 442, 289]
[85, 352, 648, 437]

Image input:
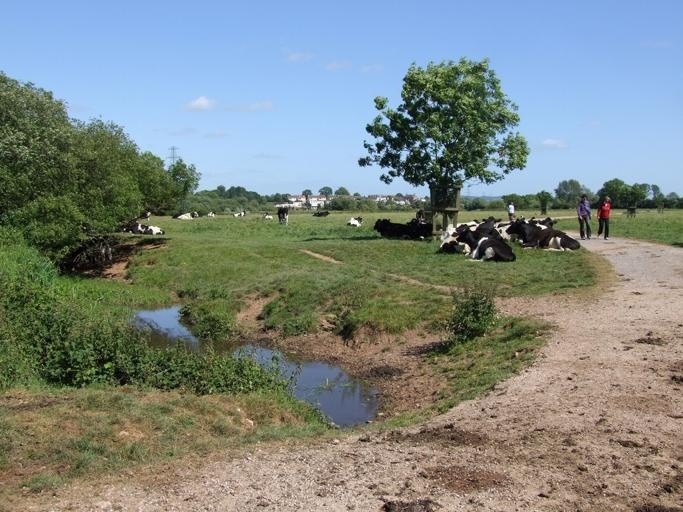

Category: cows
[346, 216, 363, 228]
[122, 223, 166, 236]
[206, 210, 215, 218]
[415, 209, 424, 219]
[373, 218, 435, 241]
[276, 207, 289, 226]
[172, 210, 200, 220]
[232, 210, 245, 218]
[435, 216, 580, 263]
[263, 213, 273, 221]
[313, 211, 329, 218]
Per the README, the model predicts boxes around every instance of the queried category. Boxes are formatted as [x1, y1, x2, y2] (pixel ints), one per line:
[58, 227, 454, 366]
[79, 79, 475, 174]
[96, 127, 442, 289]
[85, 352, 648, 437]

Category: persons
[595, 195, 611, 240]
[507, 201, 516, 223]
[575, 193, 592, 240]
[277, 206, 289, 224]
